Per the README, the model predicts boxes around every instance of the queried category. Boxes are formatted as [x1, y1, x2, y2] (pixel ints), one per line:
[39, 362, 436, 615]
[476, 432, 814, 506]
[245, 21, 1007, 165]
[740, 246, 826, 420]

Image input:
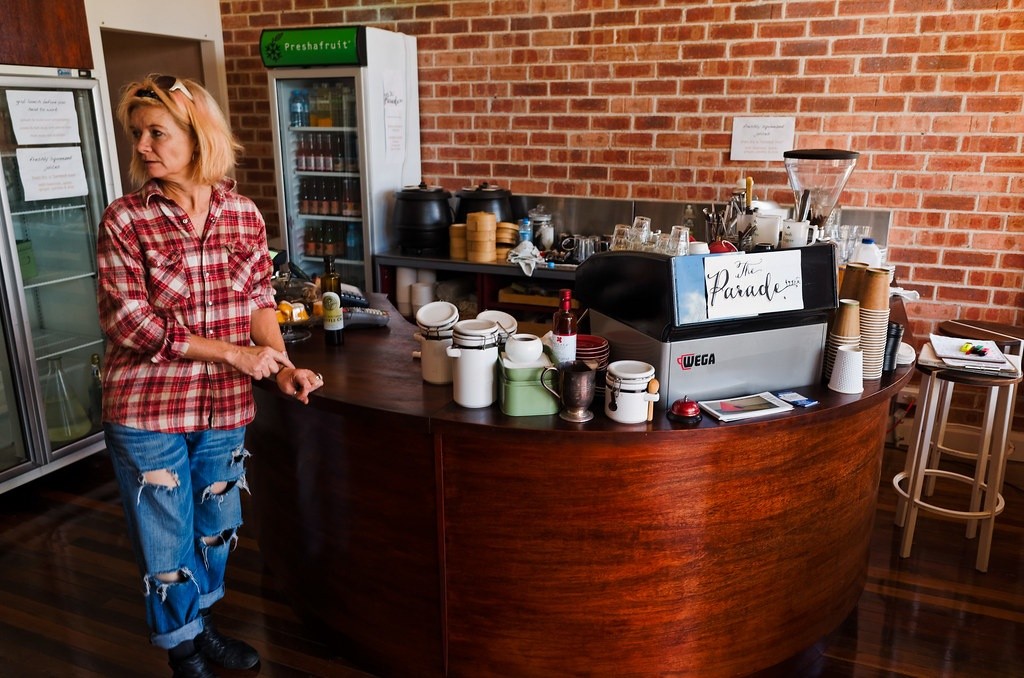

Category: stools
[893, 352, 1023, 573]
[923, 319, 1024, 498]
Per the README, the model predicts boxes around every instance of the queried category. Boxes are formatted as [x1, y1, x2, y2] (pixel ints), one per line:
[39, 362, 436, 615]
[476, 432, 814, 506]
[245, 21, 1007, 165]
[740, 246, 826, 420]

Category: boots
[168, 651, 215, 678]
[194, 609, 258, 669]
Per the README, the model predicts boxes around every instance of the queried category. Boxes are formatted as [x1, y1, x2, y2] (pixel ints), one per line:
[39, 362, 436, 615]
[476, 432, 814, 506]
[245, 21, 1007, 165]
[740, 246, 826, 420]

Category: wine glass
[541, 226, 554, 253]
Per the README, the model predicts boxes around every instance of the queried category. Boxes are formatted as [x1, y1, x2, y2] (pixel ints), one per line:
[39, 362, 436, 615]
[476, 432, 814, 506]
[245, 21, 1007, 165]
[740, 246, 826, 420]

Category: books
[918, 334, 1022, 378]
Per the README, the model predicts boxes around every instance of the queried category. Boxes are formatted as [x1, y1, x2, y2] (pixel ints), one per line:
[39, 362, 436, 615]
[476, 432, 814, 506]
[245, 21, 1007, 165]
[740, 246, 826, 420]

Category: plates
[603, 330, 658, 371]
[576, 333, 609, 397]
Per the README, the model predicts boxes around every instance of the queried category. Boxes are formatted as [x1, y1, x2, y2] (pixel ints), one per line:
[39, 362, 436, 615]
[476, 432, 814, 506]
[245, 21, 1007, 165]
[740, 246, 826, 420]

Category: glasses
[146, 73, 193, 100]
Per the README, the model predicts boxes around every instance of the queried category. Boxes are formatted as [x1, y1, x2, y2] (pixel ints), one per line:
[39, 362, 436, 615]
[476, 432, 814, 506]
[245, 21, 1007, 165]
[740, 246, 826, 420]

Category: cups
[825, 208, 904, 396]
[477, 311, 518, 352]
[446, 318, 499, 410]
[504, 334, 544, 363]
[558, 205, 818, 261]
[605, 360, 659, 424]
[415, 301, 460, 384]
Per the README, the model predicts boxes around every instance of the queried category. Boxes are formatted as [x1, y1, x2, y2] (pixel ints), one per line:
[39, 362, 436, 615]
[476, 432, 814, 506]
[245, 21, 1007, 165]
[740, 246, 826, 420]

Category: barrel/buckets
[454, 182, 513, 223]
[392, 182, 453, 259]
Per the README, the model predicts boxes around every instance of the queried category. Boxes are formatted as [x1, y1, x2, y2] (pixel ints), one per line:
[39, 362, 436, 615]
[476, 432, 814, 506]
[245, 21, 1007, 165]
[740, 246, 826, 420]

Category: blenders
[783, 149, 860, 242]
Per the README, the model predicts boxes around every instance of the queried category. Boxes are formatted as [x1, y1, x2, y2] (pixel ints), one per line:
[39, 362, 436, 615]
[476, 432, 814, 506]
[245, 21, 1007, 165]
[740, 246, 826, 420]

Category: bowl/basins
[396, 266, 437, 316]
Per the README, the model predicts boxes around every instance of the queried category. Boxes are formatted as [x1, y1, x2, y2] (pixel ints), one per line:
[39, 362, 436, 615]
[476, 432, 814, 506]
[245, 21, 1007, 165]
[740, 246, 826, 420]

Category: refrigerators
[258, 26, 423, 293]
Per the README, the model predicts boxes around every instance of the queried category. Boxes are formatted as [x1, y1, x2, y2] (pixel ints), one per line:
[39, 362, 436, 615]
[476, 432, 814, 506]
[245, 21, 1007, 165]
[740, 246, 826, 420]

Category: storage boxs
[495, 342, 560, 417]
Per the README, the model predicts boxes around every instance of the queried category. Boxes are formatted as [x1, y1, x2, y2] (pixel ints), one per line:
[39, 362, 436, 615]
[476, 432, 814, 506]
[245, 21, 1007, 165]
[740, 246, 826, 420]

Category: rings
[315, 373, 323, 380]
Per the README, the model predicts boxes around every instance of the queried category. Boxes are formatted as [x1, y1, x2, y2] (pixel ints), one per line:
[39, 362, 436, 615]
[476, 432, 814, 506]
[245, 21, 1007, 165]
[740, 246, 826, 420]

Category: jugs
[541, 361, 598, 422]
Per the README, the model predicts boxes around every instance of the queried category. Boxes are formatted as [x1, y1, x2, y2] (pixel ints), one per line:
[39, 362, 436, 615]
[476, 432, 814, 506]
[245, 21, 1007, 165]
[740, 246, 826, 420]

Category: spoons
[647, 378, 659, 421]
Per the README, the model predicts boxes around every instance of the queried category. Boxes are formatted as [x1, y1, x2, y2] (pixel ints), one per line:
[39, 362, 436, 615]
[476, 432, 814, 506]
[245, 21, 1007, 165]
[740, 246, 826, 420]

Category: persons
[96, 73, 324, 678]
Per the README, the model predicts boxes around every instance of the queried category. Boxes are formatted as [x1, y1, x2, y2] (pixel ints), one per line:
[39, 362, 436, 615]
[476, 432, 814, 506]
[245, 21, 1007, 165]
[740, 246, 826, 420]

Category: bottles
[287, 80, 366, 293]
[320, 253, 345, 350]
[552, 288, 577, 367]
[529, 203, 552, 250]
[43, 356, 92, 442]
[519, 219, 532, 244]
[87, 353, 103, 426]
[850, 239, 882, 268]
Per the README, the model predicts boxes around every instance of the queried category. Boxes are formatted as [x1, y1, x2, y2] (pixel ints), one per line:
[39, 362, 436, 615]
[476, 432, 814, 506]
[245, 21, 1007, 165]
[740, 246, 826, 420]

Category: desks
[371, 251, 579, 336]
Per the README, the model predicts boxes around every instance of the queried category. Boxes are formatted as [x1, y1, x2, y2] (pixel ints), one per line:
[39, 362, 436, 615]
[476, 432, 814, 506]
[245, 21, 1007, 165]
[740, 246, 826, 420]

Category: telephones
[303, 277, 371, 308]
